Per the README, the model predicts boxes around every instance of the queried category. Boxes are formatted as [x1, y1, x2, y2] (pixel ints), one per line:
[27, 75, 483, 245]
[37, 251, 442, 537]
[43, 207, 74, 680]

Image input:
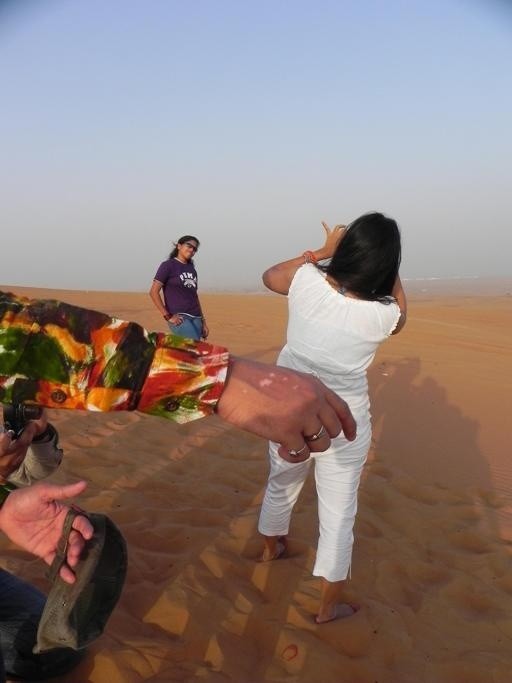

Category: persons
[261, 212, 404, 623]
[0, 288, 354, 583]
[148, 235, 209, 412]
[1, 401, 84, 681]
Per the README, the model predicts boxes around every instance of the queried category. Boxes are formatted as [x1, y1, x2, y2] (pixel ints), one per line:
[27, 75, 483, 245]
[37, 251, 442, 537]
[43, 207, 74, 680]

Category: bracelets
[163, 312, 173, 321]
[303, 250, 318, 266]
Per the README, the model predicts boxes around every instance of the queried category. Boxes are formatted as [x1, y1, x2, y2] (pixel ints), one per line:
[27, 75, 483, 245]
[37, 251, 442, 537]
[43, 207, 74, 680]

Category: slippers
[263, 542, 285, 561]
[314, 603, 354, 624]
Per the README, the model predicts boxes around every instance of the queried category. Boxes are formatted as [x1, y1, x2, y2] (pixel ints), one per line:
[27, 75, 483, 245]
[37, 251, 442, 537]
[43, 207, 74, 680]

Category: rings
[288, 442, 308, 458]
[304, 425, 326, 442]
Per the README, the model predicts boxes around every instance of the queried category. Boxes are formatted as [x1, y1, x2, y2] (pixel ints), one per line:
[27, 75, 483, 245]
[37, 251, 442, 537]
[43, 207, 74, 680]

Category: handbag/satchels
[32, 509, 128, 654]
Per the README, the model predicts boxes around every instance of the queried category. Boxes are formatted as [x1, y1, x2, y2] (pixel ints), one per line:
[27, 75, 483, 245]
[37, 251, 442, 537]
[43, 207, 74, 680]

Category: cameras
[3, 402, 43, 441]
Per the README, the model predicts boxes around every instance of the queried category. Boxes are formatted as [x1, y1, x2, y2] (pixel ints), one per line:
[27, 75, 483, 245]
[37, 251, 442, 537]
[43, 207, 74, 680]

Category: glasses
[185, 243, 197, 252]
